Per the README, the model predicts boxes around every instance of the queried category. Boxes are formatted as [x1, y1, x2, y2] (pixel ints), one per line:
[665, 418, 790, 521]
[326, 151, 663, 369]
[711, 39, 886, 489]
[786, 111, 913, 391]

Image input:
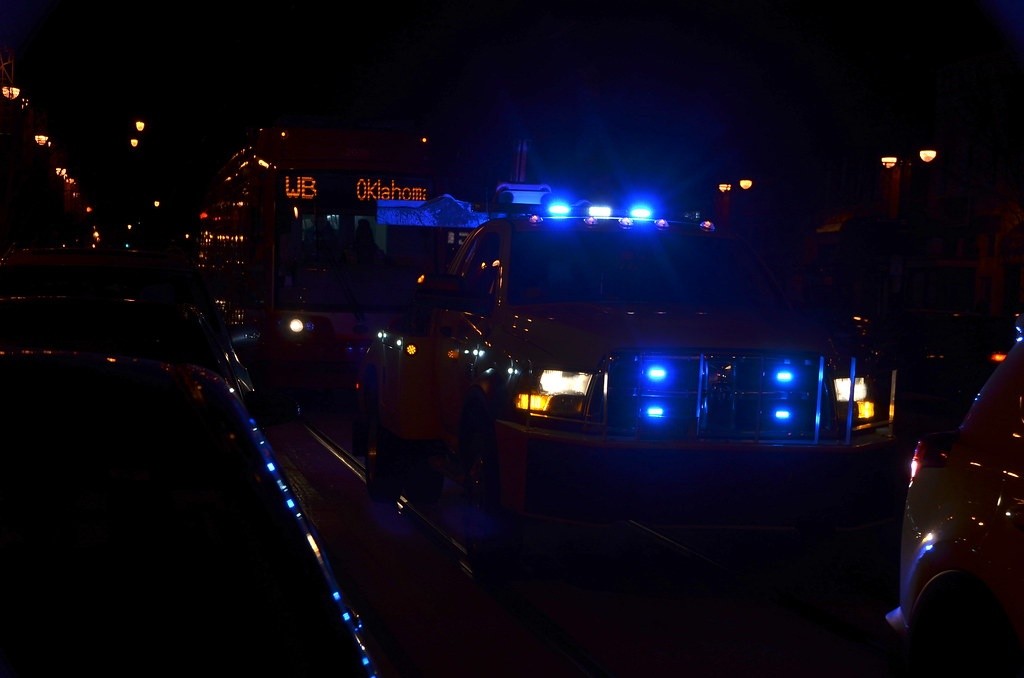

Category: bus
[194, 127, 506, 424]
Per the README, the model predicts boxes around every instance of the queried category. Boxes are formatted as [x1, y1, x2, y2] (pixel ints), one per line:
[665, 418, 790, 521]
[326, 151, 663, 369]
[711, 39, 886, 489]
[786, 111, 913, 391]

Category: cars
[349, 198, 895, 582]
[898, 334, 1024, 678]
[1, 350, 380, 678]
[1, 235, 254, 398]
